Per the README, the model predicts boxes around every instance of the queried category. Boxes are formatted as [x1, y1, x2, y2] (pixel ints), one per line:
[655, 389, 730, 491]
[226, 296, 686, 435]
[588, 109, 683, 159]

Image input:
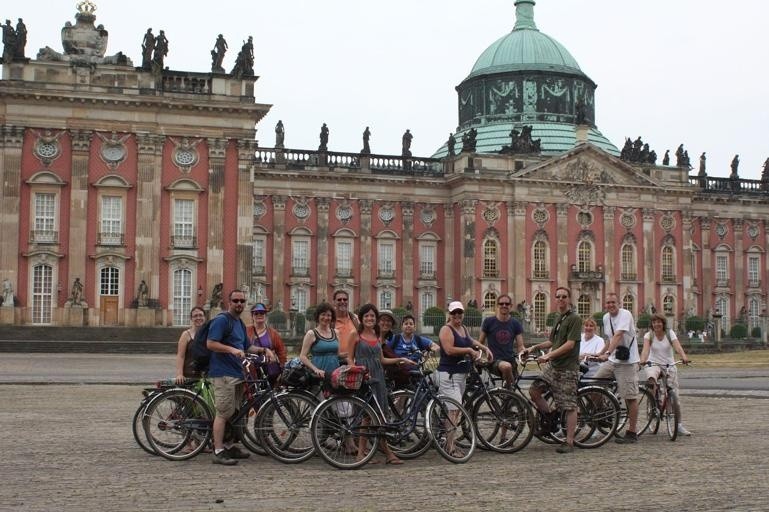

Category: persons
[332, 289, 361, 360]
[385, 315, 440, 423]
[519, 286, 582, 454]
[477, 294, 529, 448]
[345, 304, 402, 464]
[299, 304, 357, 455]
[377, 311, 402, 364]
[584, 293, 640, 444]
[432, 301, 492, 458]
[175, 305, 214, 455]
[207, 290, 252, 464]
[637, 313, 692, 436]
[241, 301, 287, 442]
[579, 319, 605, 363]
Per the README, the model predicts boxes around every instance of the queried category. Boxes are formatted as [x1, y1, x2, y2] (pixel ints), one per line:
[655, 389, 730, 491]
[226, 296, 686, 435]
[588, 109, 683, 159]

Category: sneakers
[677, 427, 691, 435]
[445, 447, 464, 460]
[456, 446, 465, 454]
[224, 446, 251, 459]
[556, 441, 573, 453]
[342, 439, 358, 454]
[212, 449, 237, 465]
[326, 438, 338, 450]
[614, 430, 637, 443]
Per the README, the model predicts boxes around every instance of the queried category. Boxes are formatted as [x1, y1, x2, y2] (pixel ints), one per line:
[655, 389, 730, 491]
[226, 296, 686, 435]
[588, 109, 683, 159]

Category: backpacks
[607, 313, 635, 361]
[253, 325, 282, 376]
[190, 312, 232, 366]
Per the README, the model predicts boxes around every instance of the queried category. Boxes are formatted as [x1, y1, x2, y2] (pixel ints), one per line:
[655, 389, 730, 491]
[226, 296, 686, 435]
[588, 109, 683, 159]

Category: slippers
[356, 456, 380, 464]
[383, 458, 404, 465]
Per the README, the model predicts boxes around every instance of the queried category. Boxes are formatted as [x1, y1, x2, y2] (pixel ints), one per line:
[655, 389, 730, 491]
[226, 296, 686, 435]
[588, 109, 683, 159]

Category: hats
[448, 301, 464, 313]
[250, 302, 268, 314]
[376, 309, 402, 330]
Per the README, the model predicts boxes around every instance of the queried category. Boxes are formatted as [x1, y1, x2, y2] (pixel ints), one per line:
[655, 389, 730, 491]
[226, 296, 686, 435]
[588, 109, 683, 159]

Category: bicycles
[309, 345, 476, 469]
[142, 346, 326, 463]
[533, 354, 656, 446]
[292, 337, 441, 459]
[133, 353, 329, 456]
[442, 341, 576, 449]
[638, 360, 692, 441]
[472, 347, 622, 454]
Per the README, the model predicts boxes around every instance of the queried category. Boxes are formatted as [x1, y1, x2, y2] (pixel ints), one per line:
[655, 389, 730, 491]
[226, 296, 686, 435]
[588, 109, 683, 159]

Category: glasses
[553, 294, 569, 299]
[230, 298, 248, 303]
[251, 311, 266, 317]
[449, 309, 464, 316]
[332, 297, 348, 302]
[496, 302, 511, 306]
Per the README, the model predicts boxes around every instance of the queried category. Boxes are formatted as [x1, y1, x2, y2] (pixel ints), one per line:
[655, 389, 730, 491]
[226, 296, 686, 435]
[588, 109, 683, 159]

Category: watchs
[604, 349, 612, 355]
